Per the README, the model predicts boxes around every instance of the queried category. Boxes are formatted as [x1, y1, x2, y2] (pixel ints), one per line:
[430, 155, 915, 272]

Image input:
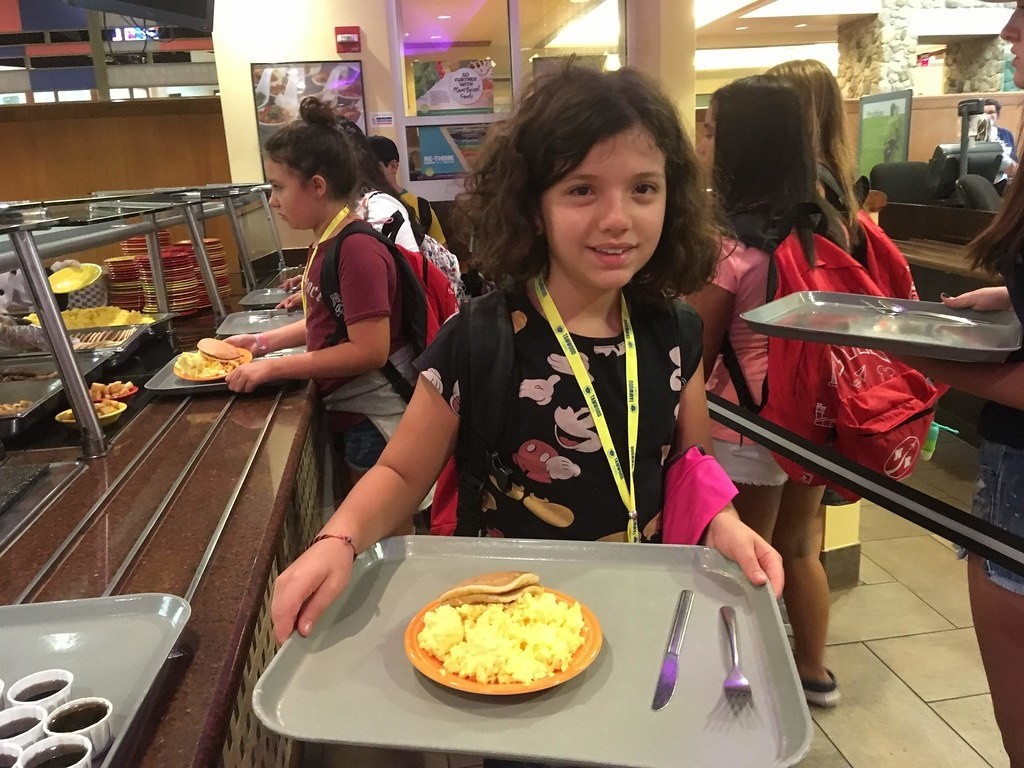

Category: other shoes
[805, 669, 844, 709]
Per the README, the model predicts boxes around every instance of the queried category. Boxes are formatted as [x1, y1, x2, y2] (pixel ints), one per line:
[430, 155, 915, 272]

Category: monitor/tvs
[68, 0, 214, 33]
[925, 142, 1002, 199]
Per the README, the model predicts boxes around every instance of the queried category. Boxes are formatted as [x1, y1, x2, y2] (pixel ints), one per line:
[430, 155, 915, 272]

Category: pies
[69, 328, 137, 349]
[197, 337, 242, 363]
[441, 573, 544, 606]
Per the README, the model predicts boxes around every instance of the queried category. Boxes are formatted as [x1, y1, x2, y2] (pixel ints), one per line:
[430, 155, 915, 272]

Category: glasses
[988, 110, 998, 114]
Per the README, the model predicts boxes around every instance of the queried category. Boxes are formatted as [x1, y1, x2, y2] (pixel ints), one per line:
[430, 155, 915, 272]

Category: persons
[940, 0, 1024, 768]
[222, 97, 430, 537]
[272, 66, 786, 647]
[335, 114, 420, 253]
[983, 99, 1018, 163]
[683, 75, 850, 704]
[367, 135, 448, 250]
[765, 58, 855, 247]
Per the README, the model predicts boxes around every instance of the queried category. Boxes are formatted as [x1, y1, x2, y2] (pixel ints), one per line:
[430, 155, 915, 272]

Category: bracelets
[304, 534, 358, 563]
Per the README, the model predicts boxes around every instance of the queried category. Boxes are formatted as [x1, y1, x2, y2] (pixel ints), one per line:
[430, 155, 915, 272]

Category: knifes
[652, 590, 694, 711]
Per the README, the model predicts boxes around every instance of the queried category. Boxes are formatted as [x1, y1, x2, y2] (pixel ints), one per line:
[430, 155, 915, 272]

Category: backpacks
[852, 206, 919, 302]
[767, 204, 938, 502]
[419, 231, 462, 298]
[320, 220, 456, 405]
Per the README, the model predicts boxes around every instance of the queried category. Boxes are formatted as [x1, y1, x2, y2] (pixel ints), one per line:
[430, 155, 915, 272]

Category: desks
[887, 237, 1004, 285]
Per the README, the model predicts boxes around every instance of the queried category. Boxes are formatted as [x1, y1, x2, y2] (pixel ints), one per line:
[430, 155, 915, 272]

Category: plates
[105, 228, 232, 313]
[48, 263, 101, 293]
[93, 387, 138, 402]
[404, 587, 602, 695]
[173, 347, 253, 381]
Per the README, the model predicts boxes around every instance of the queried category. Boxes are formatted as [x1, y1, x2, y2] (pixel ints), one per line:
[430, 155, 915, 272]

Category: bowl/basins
[258, 105, 292, 132]
[55, 402, 127, 428]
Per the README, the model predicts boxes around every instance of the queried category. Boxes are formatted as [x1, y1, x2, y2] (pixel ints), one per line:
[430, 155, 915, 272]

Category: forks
[860, 299, 977, 326]
[720, 606, 752, 698]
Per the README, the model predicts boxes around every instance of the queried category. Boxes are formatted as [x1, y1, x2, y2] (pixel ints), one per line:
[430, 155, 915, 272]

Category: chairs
[871, 161, 929, 204]
[955, 174, 1001, 212]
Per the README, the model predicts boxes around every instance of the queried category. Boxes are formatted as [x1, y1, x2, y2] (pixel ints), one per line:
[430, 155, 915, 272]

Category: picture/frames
[250, 60, 368, 199]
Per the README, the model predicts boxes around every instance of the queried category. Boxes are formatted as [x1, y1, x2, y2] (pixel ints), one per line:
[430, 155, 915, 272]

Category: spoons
[877, 299, 991, 325]
